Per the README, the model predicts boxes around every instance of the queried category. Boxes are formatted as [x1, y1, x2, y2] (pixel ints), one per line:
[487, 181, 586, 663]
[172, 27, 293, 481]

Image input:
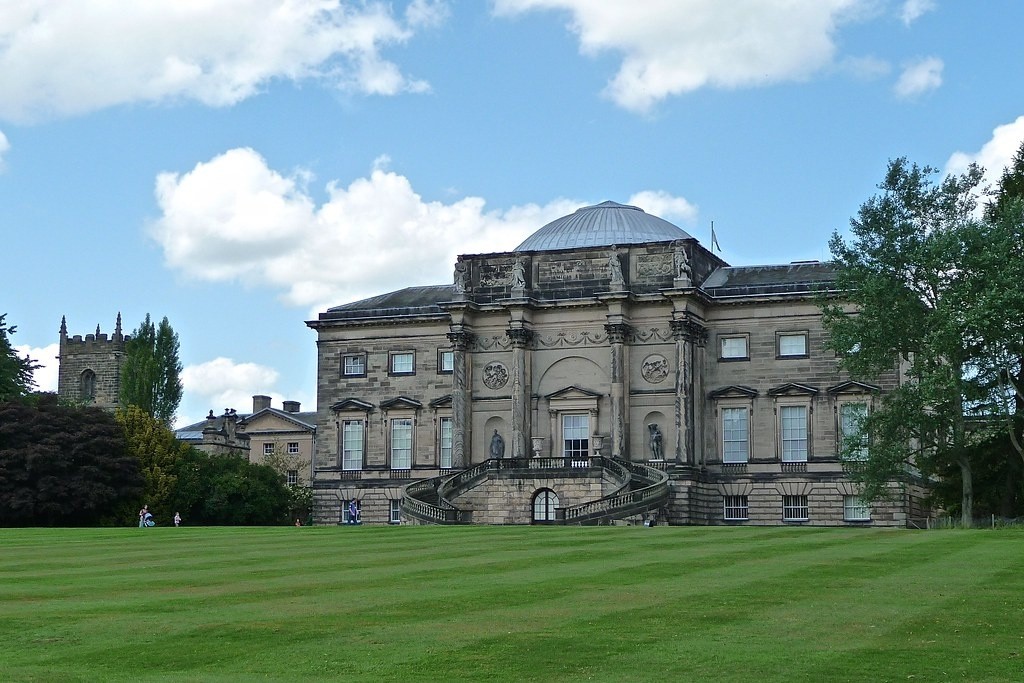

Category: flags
[710, 225, 724, 253]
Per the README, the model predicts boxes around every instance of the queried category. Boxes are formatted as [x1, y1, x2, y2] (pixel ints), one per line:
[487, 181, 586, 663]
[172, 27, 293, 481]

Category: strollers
[142, 513, 155, 527]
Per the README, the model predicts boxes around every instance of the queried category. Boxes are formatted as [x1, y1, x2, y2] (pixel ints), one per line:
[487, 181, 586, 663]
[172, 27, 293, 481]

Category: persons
[346, 497, 360, 526]
[453, 254, 468, 289]
[295, 518, 302, 528]
[605, 245, 627, 286]
[488, 428, 505, 464]
[138, 504, 155, 528]
[648, 423, 664, 460]
[508, 251, 527, 293]
[174, 512, 182, 528]
[669, 238, 691, 282]
